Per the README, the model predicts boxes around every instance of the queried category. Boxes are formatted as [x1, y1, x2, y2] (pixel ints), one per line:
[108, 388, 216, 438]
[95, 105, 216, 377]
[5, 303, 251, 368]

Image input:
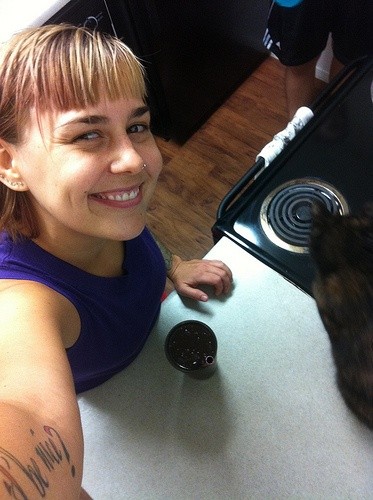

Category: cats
[310, 200, 372, 432]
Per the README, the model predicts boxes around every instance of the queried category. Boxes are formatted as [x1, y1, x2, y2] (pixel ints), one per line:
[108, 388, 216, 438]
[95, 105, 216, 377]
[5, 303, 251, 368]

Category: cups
[164, 320, 218, 379]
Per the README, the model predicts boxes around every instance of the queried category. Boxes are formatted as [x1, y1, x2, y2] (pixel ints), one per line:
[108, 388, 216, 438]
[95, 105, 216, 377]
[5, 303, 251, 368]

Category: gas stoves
[210, 61, 372, 298]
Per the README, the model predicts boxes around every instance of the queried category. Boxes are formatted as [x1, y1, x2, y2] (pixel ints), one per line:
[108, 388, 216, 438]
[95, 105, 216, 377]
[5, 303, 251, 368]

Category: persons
[262, 0, 373, 123]
[0, 22, 234, 499]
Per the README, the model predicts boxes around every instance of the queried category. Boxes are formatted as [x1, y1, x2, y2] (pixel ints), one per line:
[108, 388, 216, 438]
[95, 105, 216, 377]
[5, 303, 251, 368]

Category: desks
[75, 236, 373, 499]
